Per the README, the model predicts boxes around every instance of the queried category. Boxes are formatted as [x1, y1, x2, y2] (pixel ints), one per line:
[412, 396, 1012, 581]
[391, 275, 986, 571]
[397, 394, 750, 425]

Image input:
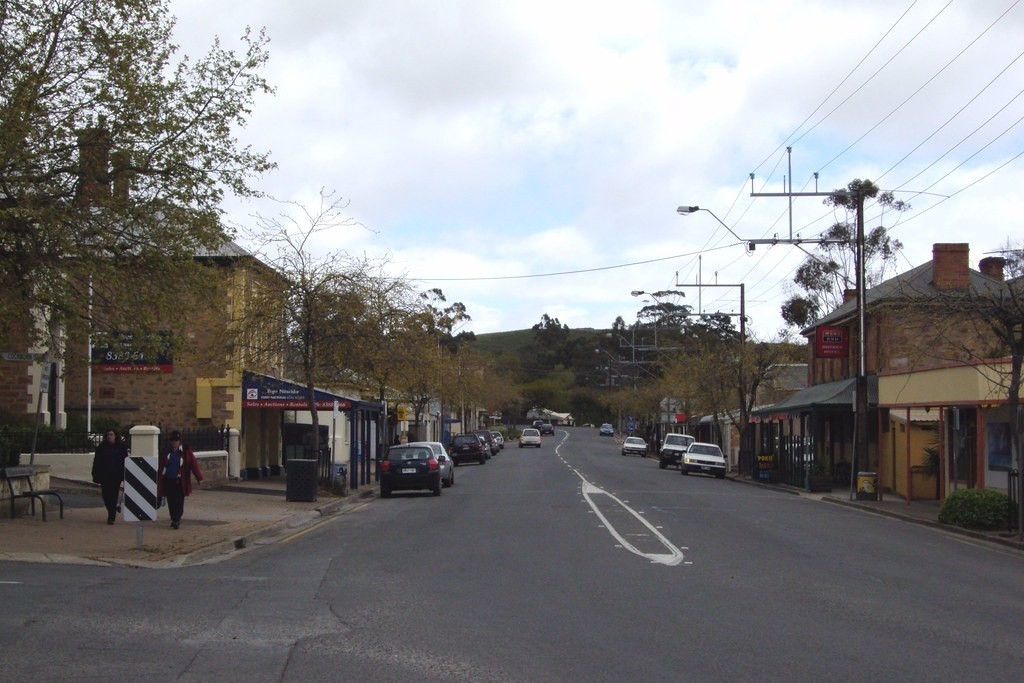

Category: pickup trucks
[519, 429, 541, 448]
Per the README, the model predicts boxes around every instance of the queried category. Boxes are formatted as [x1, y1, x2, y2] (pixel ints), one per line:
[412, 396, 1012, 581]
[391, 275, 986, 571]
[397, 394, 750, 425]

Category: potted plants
[808, 463, 823, 492]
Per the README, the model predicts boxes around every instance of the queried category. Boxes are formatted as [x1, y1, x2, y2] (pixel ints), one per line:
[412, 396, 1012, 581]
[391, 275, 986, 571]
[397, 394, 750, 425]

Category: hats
[169, 430, 184, 441]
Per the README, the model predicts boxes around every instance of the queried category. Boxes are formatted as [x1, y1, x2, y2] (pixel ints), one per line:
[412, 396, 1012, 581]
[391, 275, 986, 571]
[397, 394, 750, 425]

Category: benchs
[4, 464, 63, 522]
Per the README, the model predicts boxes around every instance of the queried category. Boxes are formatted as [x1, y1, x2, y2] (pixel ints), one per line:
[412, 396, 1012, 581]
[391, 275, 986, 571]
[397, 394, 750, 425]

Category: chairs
[418, 451, 426, 459]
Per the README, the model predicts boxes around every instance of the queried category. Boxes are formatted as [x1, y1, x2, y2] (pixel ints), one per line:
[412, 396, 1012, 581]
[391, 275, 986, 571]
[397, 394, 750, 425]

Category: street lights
[586, 349, 660, 453]
[606, 333, 693, 436]
[631, 291, 750, 476]
[677, 204, 868, 470]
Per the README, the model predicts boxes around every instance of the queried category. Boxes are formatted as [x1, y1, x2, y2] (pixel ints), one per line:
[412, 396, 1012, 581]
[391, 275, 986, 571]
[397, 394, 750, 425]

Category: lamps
[429, 411, 441, 420]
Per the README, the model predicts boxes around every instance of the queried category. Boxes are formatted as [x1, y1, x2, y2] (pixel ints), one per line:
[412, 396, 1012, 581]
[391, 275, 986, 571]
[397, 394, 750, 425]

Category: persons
[158, 429, 202, 530]
[91, 429, 128, 524]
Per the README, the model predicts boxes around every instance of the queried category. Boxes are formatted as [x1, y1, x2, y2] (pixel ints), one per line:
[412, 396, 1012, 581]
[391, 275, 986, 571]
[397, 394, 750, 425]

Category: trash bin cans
[856, 471, 877, 501]
[285, 458, 319, 502]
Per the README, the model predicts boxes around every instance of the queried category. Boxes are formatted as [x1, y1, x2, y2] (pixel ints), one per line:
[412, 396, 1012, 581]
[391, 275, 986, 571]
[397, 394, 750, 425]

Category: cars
[531, 421, 543, 430]
[681, 442, 728, 479]
[379, 445, 445, 499]
[401, 442, 454, 488]
[786, 443, 814, 469]
[541, 424, 554, 436]
[622, 437, 648, 457]
[448, 434, 486, 467]
[600, 424, 614, 437]
[659, 433, 695, 468]
[468, 430, 505, 459]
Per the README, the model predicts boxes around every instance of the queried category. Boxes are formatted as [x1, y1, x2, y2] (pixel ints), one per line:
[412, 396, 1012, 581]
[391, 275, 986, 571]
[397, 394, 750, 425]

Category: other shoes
[108, 515, 115, 525]
[171, 522, 179, 528]
